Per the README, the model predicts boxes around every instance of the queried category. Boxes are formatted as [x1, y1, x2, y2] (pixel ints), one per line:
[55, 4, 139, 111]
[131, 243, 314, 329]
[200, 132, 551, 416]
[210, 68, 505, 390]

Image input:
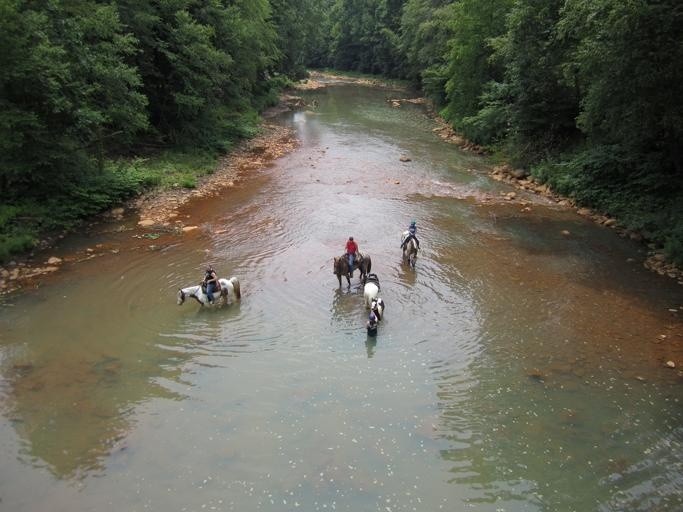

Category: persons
[366, 312, 378, 336]
[202, 264, 217, 305]
[344, 237, 358, 278]
[398, 219, 421, 250]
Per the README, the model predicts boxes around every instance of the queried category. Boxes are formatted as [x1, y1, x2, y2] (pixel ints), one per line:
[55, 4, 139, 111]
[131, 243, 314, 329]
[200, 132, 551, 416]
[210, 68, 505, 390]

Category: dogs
[371, 297, 385, 320]
[177, 276, 241, 306]
[363, 273, 380, 309]
[400, 230, 419, 266]
[333, 252, 371, 286]
